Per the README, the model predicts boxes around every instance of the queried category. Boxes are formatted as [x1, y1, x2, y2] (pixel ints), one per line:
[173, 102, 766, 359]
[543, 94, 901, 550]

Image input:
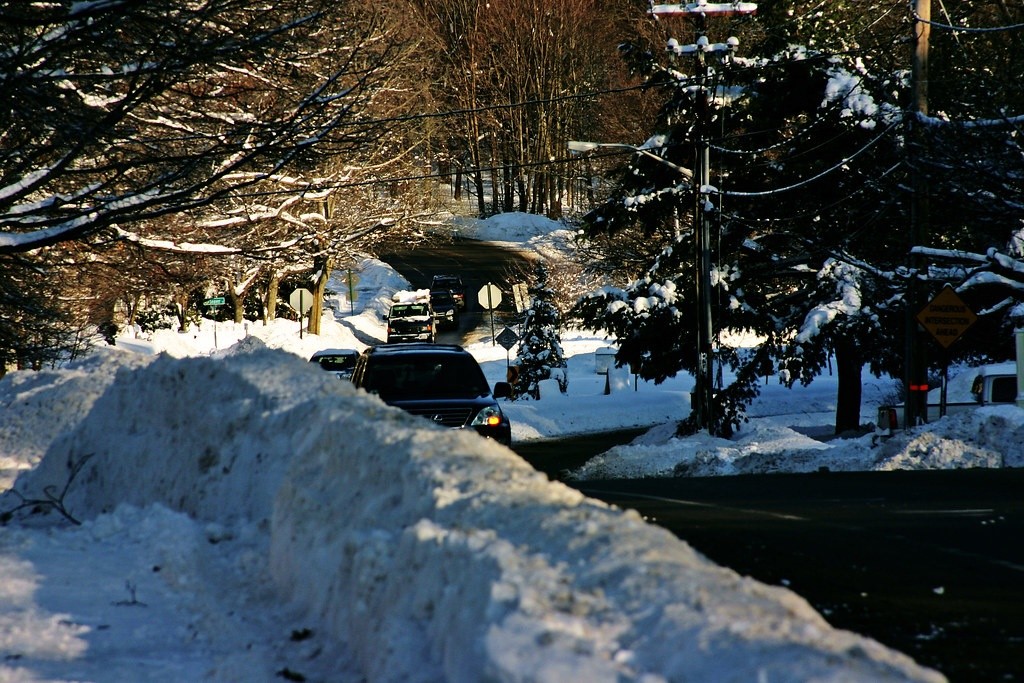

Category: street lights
[567, 140, 715, 436]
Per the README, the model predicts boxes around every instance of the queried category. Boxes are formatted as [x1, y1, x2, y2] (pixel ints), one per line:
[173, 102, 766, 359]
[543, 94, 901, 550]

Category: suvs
[429, 273, 469, 312]
[349, 341, 511, 448]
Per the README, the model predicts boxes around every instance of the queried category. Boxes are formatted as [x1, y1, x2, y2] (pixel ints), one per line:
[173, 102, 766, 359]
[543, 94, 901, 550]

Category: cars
[309, 348, 360, 381]
[430, 290, 460, 332]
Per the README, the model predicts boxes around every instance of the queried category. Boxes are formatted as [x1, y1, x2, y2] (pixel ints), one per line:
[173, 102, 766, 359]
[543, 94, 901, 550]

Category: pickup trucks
[875, 359, 1017, 442]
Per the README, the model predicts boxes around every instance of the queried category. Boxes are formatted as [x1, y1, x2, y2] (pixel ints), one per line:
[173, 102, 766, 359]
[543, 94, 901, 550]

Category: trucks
[382, 300, 437, 343]
